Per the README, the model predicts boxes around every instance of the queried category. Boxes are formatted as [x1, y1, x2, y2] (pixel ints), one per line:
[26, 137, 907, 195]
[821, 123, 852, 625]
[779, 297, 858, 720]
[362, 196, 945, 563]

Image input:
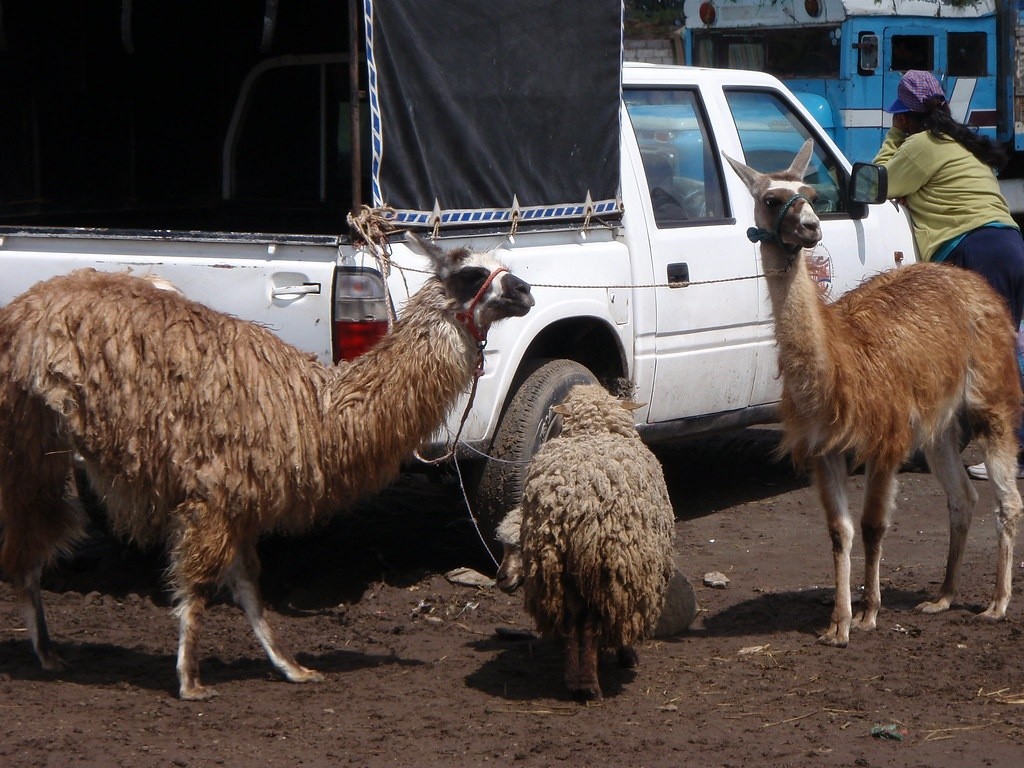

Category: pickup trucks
[0, 61, 924, 572]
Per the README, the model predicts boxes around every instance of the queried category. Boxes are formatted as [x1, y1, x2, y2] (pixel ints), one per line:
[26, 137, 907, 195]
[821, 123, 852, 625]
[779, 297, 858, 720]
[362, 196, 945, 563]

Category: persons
[871, 70, 1024, 480]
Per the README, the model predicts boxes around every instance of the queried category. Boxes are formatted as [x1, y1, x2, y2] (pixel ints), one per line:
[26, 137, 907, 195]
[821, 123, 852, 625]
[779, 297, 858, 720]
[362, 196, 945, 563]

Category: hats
[885, 70, 945, 113]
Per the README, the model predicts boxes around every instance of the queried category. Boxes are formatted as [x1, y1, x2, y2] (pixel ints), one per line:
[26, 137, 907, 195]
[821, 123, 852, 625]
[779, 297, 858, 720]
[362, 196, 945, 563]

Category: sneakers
[967, 462, 1024, 479]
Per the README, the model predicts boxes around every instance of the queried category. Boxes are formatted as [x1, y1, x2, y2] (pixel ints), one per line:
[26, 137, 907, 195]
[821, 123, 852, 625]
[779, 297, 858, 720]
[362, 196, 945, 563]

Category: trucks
[625, 0, 1024, 220]
